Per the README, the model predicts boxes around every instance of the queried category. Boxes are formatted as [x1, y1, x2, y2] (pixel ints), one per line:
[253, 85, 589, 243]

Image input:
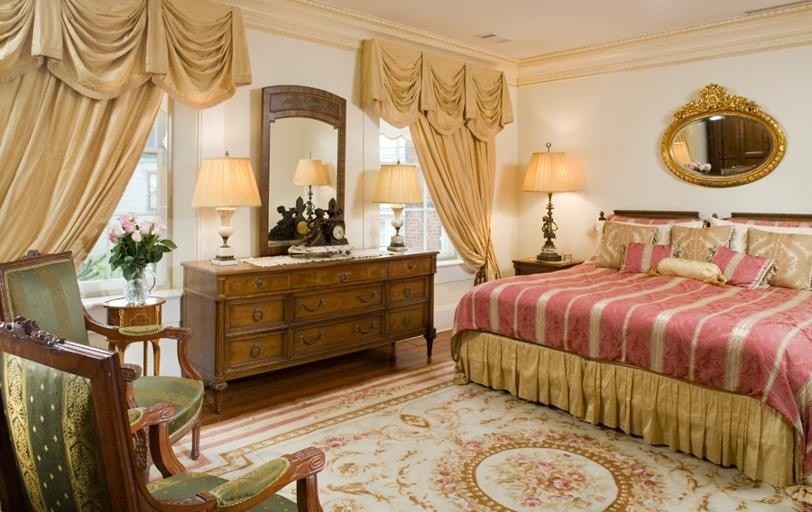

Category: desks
[101, 295, 168, 376]
[178, 248, 442, 414]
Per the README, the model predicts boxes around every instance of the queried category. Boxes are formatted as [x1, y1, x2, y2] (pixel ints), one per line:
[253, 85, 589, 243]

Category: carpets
[148, 360, 811, 511]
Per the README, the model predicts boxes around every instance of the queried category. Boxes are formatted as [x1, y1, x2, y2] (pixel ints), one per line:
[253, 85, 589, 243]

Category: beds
[448, 207, 812, 489]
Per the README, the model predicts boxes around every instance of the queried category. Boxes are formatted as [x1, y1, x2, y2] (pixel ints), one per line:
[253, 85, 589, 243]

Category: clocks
[307, 195, 350, 248]
[266, 195, 312, 243]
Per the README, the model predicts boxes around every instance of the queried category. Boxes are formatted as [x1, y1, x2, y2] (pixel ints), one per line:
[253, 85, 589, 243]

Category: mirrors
[658, 80, 789, 191]
[258, 83, 347, 258]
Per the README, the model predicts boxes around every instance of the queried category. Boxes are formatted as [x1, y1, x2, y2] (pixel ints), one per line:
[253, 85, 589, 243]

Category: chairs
[0, 312, 328, 512]
[0, 247, 208, 462]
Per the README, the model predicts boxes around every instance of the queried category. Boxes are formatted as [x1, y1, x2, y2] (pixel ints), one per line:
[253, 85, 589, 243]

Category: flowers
[688, 160, 712, 175]
[106, 210, 179, 297]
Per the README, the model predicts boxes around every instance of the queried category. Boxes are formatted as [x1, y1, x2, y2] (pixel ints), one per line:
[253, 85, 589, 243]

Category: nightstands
[511, 256, 584, 276]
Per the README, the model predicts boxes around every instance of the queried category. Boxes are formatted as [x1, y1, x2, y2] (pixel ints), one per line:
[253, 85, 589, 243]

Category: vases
[123, 260, 158, 306]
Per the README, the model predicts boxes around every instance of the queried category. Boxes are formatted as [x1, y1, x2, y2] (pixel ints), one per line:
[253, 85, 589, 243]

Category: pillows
[581, 211, 812, 292]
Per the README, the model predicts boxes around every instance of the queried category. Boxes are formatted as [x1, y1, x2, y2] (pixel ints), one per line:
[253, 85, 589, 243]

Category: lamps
[519, 140, 585, 262]
[292, 151, 330, 223]
[672, 140, 691, 169]
[370, 159, 427, 252]
[189, 144, 266, 267]
